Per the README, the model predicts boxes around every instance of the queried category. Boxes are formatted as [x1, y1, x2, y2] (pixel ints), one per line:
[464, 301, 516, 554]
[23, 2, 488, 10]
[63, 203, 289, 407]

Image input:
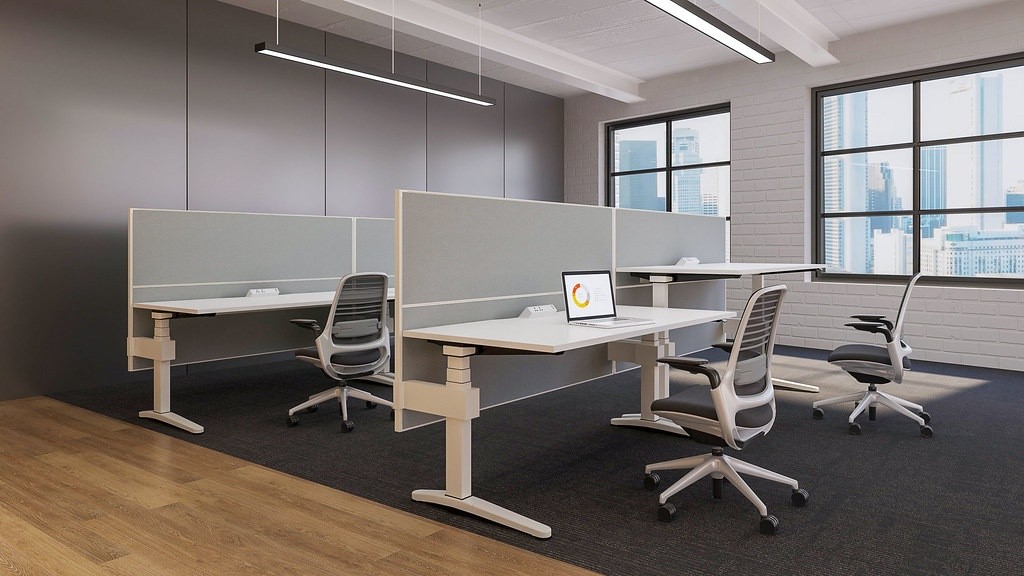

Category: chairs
[810, 271, 938, 437]
[287, 271, 395, 435]
[642, 282, 810, 537]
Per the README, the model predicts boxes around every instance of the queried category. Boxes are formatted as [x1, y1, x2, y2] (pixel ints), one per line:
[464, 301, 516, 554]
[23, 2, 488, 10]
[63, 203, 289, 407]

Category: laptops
[561, 270, 656, 329]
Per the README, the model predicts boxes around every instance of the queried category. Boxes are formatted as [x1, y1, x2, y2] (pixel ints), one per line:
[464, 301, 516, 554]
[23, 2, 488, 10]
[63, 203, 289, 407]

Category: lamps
[254, 0, 497, 108]
[646, 0, 776, 66]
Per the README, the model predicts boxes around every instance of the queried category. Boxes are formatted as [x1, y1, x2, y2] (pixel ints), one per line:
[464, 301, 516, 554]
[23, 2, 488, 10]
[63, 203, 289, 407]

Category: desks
[0, 394, 609, 576]
[406, 303, 738, 540]
[608, 259, 828, 439]
[135, 288, 395, 440]
